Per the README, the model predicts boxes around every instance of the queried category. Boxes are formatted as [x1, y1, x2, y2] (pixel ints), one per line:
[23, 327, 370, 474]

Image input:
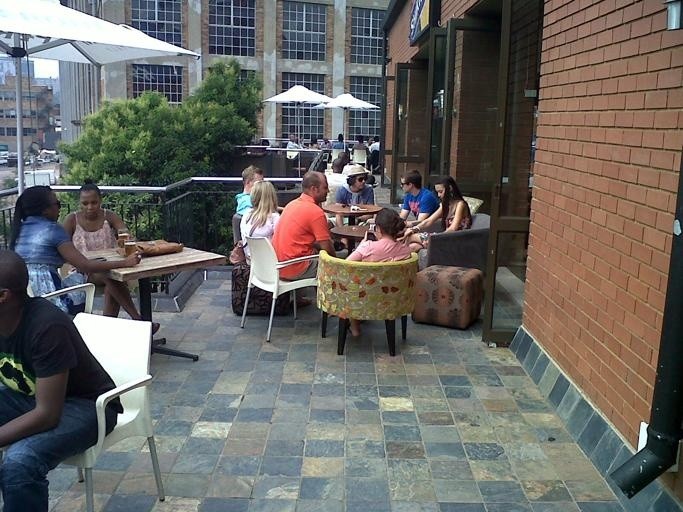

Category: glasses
[358, 177, 367, 182]
[400, 179, 407, 185]
[50, 201, 61, 209]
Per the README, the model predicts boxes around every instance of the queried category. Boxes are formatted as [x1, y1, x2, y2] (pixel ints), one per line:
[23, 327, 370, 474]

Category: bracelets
[411, 225, 420, 233]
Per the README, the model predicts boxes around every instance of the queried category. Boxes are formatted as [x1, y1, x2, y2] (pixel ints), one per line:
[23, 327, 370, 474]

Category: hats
[347, 166, 367, 177]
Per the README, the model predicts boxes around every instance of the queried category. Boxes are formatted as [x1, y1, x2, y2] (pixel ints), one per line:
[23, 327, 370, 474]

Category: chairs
[53, 309, 167, 512]
[330, 146, 369, 172]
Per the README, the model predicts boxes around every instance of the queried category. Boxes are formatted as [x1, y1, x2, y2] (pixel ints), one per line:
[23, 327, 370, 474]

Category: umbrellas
[0, 1, 200, 197]
[257, 83, 333, 150]
[314, 92, 381, 159]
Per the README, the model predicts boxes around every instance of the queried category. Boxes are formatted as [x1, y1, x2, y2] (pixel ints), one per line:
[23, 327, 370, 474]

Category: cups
[118, 229, 129, 247]
[125, 241, 136, 260]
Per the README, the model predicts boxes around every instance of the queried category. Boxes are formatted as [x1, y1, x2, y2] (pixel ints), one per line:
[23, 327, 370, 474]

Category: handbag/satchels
[116, 240, 184, 258]
[229, 240, 246, 264]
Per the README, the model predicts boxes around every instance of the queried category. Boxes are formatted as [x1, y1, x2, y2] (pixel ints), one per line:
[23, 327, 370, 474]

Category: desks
[77, 238, 227, 362]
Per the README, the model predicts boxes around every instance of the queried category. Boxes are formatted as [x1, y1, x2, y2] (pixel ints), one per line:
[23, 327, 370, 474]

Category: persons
[402, 176, 471, 256]
[342, 208, 414, 338]
[0, 248, 125, 511]
[284, 130, 385, 179]
[11, 186, 143, 334]
[239, 179, 315, 311]
[273, 171, 339, 298]
[330, 163, 376, 235]
[233, 164, 266, 221]
[61, 178, 160, 342]
[398, 168, 440, 223]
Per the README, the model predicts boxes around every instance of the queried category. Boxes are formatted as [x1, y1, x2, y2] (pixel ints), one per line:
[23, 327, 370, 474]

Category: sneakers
[152, 323, 160, 335]
